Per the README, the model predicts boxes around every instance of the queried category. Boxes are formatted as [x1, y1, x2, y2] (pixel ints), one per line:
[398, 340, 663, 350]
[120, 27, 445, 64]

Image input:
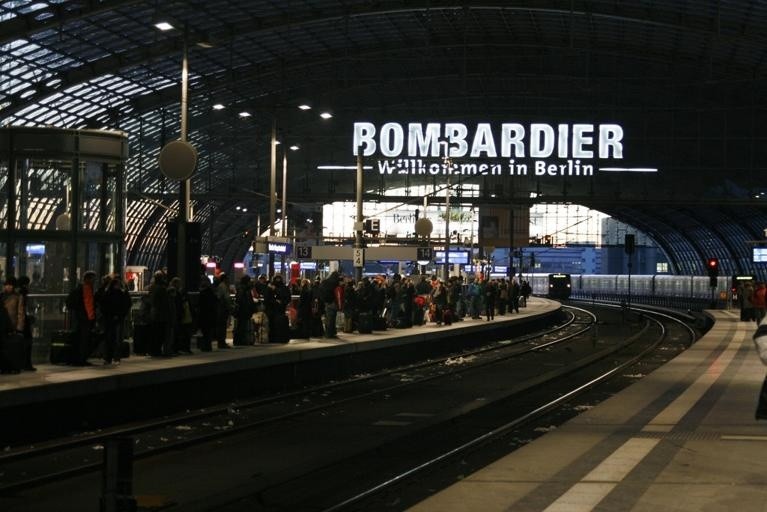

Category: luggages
[0, 329, 25, 377]
[50, 295, 527, 367]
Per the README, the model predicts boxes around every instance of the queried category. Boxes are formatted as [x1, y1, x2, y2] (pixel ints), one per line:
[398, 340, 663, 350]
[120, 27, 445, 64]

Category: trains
[500, 274, 572, 299]
[570, 273, 755, 296]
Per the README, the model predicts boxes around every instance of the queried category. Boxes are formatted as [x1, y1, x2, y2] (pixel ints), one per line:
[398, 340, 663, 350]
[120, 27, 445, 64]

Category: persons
[234, 274, 291, 347]
[751, 312, 766, 420]
[750, 281, 766, 321]
[141, 270, 239, 361]
[741, 282, 753, 321]
[293, 269, 531, 340]
[1, 275, 37, 374]
[32, 271, 134, 368]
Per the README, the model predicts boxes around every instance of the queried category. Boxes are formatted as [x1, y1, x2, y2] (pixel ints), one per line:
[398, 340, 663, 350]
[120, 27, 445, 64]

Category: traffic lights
[708, 257, 719, 278]
